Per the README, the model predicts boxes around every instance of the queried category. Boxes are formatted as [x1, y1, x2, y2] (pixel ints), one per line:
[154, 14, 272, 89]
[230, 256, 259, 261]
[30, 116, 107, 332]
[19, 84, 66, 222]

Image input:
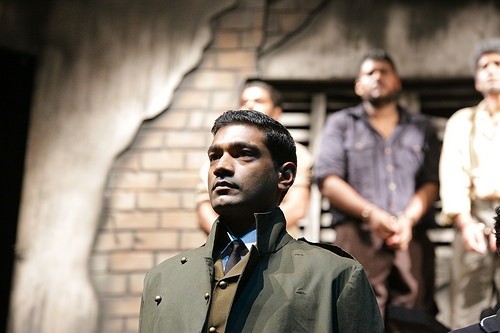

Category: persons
[196, 82, 313, 240]
[439, 42, 500, 330]
[140, 110, 384, 332]
[314, 50, 441, 333]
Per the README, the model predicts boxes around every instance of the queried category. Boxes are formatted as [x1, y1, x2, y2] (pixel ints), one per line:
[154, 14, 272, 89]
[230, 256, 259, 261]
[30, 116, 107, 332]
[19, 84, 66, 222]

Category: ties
[225, 239, 244, 276]
